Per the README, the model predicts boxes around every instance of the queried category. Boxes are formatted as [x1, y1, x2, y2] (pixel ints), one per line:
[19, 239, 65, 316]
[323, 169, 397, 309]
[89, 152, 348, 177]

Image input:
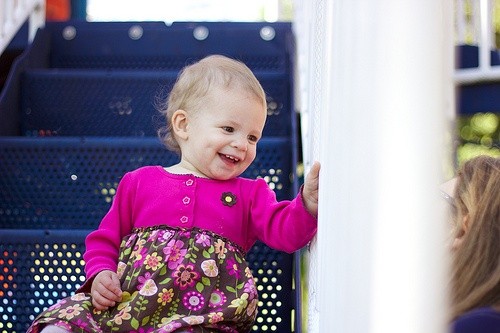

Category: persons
[28, 49, 320, 333]
[443, 154, 500, 333]
